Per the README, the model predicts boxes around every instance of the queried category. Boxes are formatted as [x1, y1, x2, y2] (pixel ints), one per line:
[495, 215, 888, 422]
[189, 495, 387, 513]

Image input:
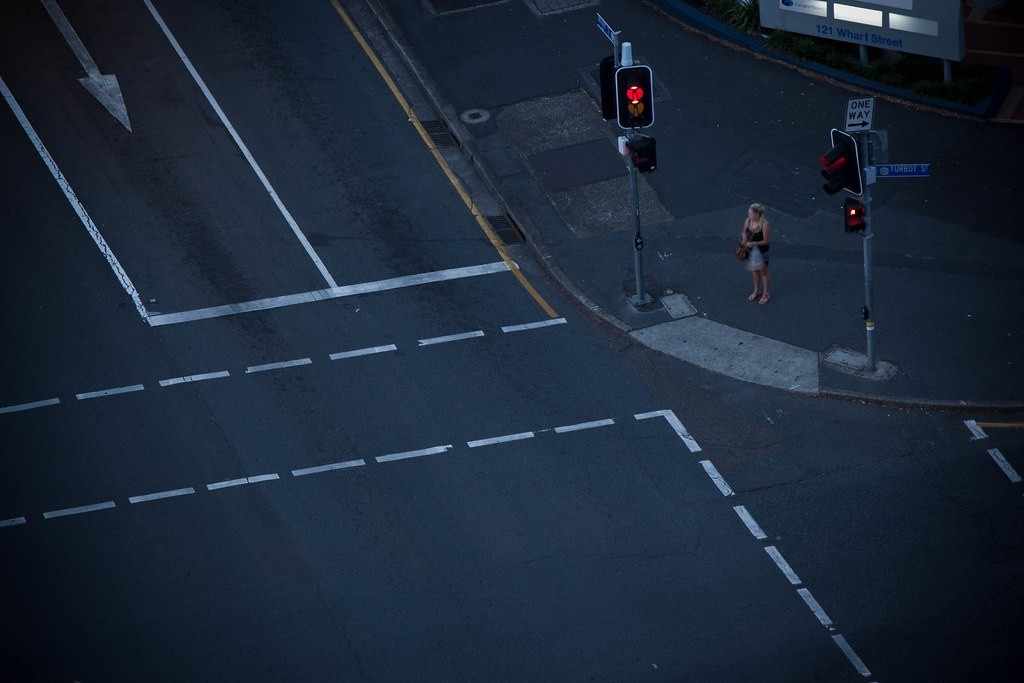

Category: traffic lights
[844, 198, 867, 234]
[628, 134, 657, 171]
[819, 129, 862, 197]
[617, 65, 655, 129]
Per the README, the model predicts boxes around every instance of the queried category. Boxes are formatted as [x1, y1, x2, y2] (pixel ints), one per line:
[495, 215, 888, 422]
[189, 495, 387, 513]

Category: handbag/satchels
[746, 246, 765, 270]
[735, 241, 746, 259]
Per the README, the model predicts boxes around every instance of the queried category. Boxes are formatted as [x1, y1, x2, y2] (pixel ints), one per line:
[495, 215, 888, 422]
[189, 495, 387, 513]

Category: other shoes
[748, 288, 762, 301]
[759, 291, 771, 304]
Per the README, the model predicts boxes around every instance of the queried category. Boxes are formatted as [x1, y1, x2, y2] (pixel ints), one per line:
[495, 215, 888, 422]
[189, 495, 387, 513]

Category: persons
[740, 202, 774, 306]
